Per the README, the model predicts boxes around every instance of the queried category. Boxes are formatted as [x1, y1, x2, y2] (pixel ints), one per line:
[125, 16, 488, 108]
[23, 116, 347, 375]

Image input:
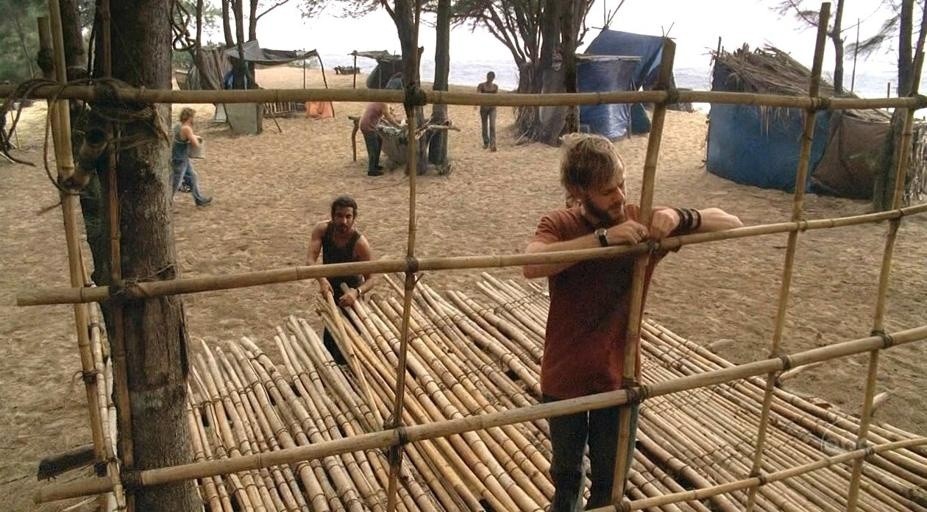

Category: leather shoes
[368, 171, 384, 176]
[375, 165, 383, 169]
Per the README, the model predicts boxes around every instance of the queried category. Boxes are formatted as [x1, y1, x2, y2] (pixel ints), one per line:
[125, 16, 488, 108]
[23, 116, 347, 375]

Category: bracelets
[668, 205, 701, 237]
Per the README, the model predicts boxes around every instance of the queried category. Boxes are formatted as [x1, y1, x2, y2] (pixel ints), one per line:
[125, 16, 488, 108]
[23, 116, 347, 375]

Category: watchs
[355, 287, 362, 298]
[594, 227, 609, 247]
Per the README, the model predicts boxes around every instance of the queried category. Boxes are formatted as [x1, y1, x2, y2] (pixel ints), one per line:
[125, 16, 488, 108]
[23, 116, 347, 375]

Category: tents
[576, 29, 676, 141]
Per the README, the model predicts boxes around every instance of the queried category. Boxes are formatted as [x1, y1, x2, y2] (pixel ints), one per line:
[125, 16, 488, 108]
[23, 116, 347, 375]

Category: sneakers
[196, 195, 212, 205]
[491, 146, 496, 151]
[482, 143, 488, 148]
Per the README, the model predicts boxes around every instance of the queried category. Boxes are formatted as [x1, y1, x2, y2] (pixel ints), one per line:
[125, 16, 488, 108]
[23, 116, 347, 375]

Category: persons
[360, 102, 403, 176]
[304, 195, 378, 367]
[472, 71, 498, 151]
[522, 133, 738, 510]
[171, 107, 212, 207]
